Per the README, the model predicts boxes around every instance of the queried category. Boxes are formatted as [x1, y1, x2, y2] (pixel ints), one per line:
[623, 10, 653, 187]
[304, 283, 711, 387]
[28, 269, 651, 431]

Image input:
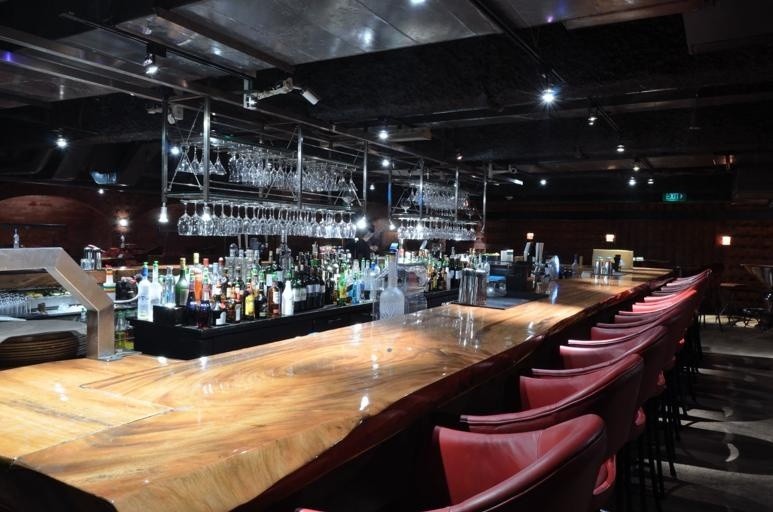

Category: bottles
[569, 254, 583, 276]
[79, 244, 490, 353]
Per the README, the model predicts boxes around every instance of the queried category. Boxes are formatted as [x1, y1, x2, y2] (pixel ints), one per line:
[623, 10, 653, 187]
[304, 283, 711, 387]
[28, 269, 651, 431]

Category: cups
[593, 259, 612, 275]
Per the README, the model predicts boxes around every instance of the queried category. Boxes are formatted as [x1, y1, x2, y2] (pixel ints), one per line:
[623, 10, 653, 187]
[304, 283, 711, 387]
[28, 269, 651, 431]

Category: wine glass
[175, 136, 479, 241]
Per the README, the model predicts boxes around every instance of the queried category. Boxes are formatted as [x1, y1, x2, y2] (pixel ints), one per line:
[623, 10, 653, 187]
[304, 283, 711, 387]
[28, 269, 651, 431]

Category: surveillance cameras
[300, 86, 321, 106]
